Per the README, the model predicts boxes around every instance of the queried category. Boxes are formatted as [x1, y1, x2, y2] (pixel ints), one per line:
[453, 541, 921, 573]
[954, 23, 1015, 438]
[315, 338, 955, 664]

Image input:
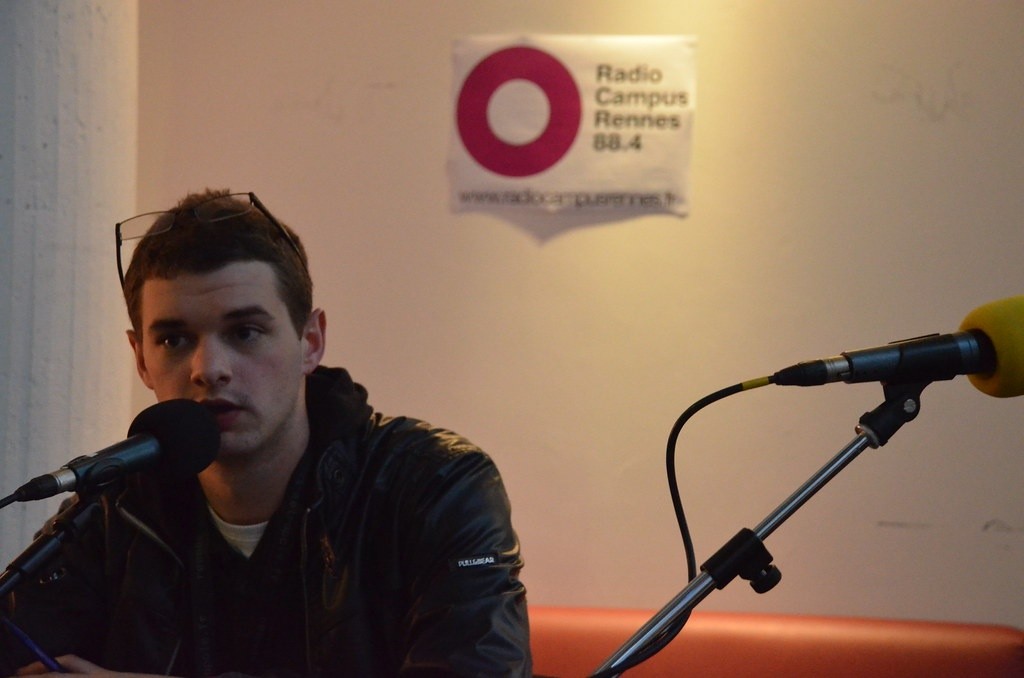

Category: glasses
[115, 192, 313, 293]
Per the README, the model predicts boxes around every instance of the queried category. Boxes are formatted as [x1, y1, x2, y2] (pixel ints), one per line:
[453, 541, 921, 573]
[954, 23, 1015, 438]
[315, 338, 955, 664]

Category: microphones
[775, 293, 1024, 398]
[17, 398, 222, 503]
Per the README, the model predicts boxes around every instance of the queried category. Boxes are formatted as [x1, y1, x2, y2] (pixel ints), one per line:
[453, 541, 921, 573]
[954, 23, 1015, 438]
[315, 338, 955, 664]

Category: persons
[0, 187, 533, 678]
